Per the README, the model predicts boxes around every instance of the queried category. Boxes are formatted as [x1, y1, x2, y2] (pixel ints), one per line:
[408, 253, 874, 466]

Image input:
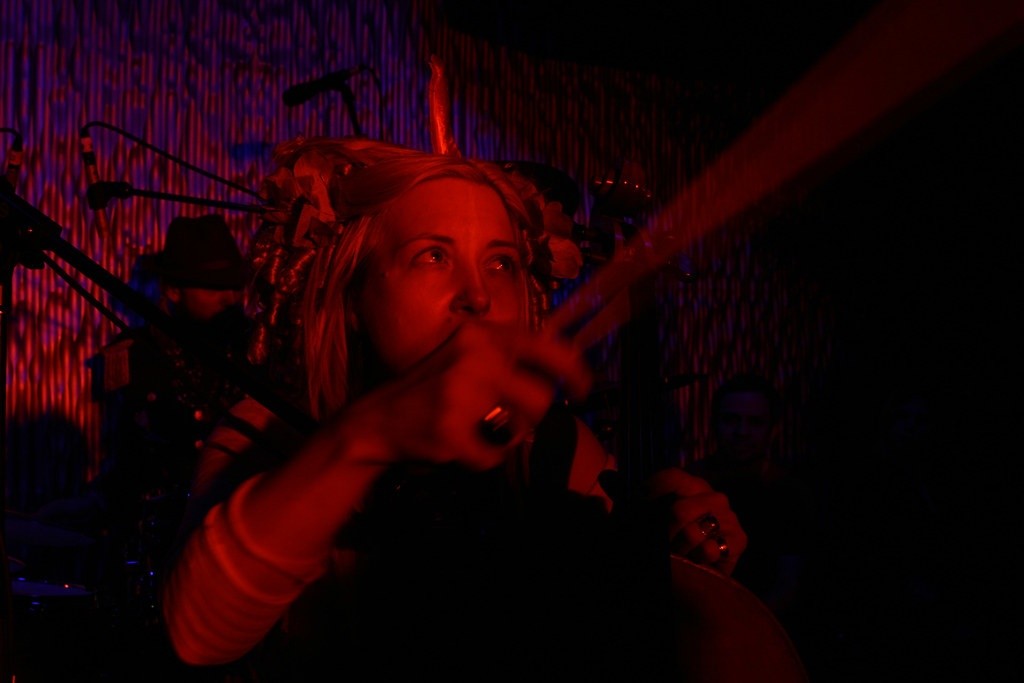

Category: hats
[133, 215, 251, 288]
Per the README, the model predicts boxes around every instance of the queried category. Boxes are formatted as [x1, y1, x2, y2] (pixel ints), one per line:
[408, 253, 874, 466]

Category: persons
[682, 373, 812, 632]
[158, 132, 749, 683]
[86, 215, 297, 628]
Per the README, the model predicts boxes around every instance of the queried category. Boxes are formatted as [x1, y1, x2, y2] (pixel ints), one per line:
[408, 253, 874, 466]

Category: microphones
[282, 63, 366, 107]
[4, 134, 24, 195]
[77, 127, 110, 237]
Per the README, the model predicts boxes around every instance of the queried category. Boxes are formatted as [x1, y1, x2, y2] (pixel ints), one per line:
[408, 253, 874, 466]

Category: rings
[697, 512, 720, 538]
[480, 404, 514, 444]
[709, 536, 729, 559]
